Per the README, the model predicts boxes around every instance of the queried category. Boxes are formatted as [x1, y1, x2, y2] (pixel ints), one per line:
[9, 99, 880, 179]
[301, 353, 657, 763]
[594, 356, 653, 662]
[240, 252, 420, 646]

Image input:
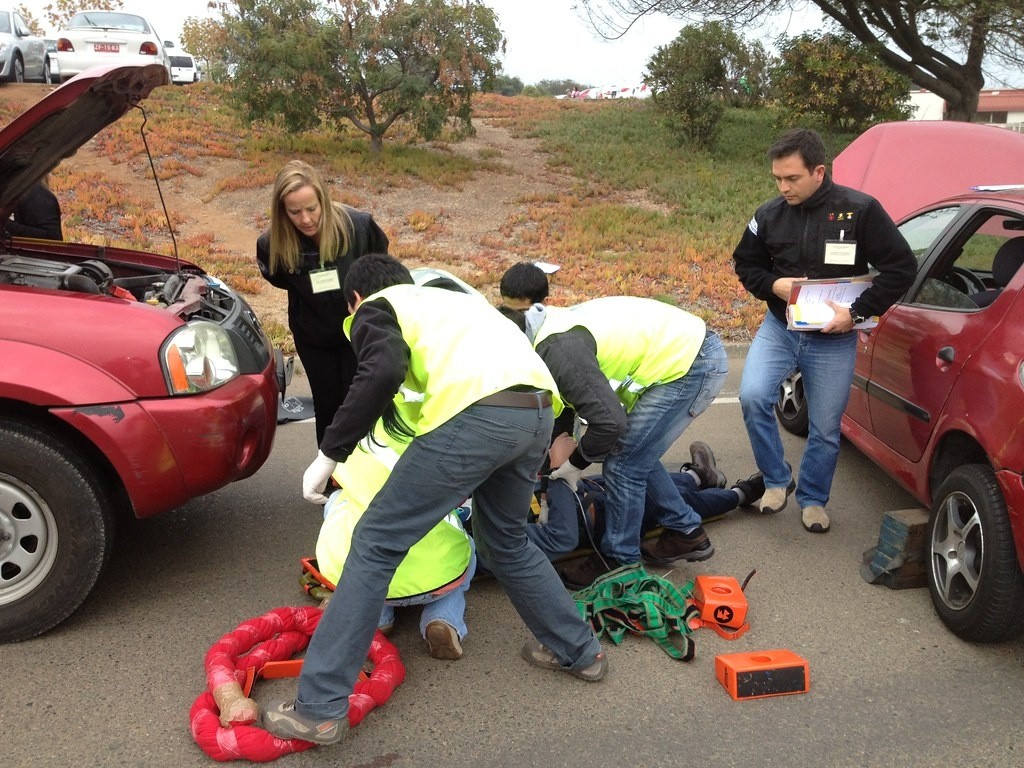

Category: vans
[167, 52, 202, 84]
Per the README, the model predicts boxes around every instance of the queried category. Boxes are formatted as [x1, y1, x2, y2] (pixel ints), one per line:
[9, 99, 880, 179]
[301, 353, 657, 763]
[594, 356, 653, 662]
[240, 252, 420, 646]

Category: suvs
[0, 52, 293, 643]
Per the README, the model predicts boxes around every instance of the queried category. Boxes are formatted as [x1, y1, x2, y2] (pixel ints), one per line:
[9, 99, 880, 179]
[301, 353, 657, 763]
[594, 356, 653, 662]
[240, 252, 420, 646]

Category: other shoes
[378, 621, 394, 632]
[680, 440, 727, 488]
[426, 620, 463, 661]
[731, 459, 793, 508]
[801, 505, 830, 532]
[639, 525, 715, 565]
[759, 476, 797, 514]
[560, 551, 644, 590]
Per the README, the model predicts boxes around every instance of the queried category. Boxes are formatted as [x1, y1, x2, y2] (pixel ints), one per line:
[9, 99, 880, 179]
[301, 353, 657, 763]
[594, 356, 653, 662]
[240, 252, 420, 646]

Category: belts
[478, 389, 556, 408]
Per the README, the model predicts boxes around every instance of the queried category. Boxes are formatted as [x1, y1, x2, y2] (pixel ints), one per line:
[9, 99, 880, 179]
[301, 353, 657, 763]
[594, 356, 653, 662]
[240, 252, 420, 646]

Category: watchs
[848, 307, 867, 324]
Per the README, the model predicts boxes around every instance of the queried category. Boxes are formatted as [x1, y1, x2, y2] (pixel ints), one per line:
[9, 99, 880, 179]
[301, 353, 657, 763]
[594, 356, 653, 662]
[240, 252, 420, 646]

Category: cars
[41, 38, 60, 80]
[55, 9, 174, 84]
[774, 120, 1024, 641]
[0, 6, 52, 85]
[554, 83, 667, 100]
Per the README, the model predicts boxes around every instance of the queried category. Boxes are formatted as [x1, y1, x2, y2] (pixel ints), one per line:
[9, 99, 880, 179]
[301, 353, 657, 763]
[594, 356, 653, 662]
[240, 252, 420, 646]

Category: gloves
[302, 449, 338, 505]
[547, 460, 584, 493]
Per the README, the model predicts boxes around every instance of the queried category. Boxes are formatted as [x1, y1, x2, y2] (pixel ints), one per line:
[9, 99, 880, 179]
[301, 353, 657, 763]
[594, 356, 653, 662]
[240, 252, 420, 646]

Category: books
[784, 273, 883, 335]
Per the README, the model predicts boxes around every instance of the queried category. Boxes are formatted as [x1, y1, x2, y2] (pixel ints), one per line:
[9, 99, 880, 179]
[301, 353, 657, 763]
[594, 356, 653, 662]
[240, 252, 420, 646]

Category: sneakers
[521, 639, 610, 681]
[259, 701, 349, 746]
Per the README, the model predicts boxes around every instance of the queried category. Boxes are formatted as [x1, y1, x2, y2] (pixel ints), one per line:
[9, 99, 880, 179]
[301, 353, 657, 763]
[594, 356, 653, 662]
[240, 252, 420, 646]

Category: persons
[0, 149, 77, 241]
[261, 253, 608, 744]
[255, 159, 793, 748]
[732, 128, 921, 534]
